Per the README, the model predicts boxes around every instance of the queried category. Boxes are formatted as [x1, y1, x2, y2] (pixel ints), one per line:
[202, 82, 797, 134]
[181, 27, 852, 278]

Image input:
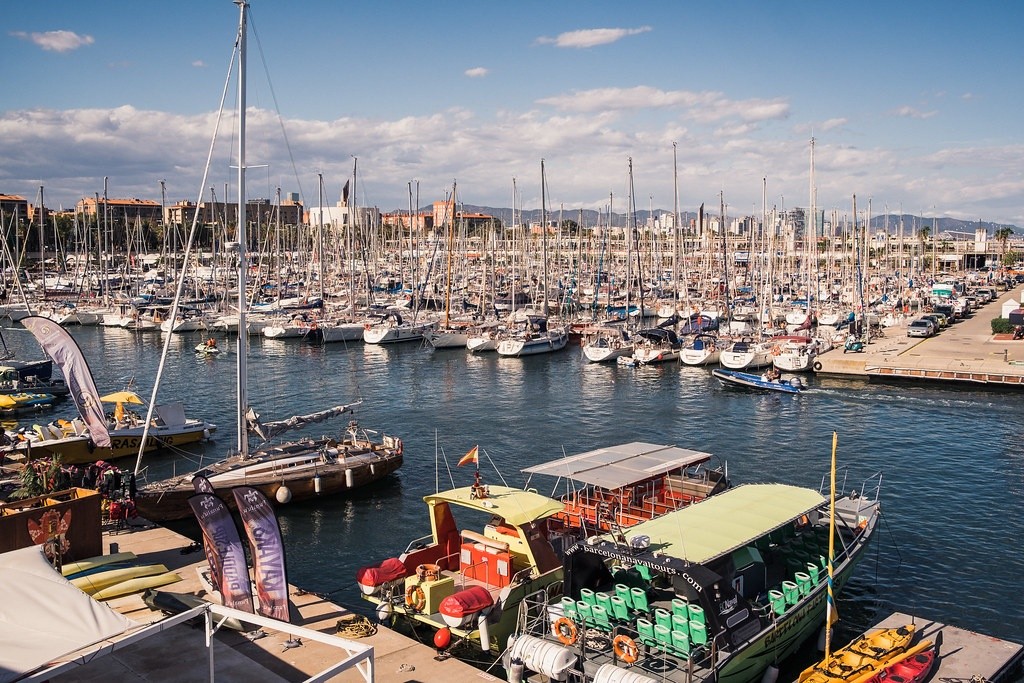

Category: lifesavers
[814, 361, 821, 370]
[415, 563, 440, 582]
[612, 634, 639, 664]
[554, 617, 577, 646]
[364, 323, 370, 329]
[311, 323, 317, 330]
[405, 585, 425, 610]
[581, 339, 585, 346]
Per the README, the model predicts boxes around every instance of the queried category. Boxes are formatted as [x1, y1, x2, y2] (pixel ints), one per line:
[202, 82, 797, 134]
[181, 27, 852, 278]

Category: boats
[501, 466, 884, 683]
[0, 384, 220, 467]
[0, 393, 56, 417]
[354, 427, 732, 668]
[862, 650, 935, 683]
[712, 366, 801, 396]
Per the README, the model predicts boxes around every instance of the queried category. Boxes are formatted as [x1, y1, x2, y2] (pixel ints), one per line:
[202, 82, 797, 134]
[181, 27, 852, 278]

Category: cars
[904, 264, 1024, 339]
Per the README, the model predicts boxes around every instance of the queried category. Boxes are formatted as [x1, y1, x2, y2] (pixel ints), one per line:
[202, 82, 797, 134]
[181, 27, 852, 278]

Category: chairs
[561, 519, 852, 658]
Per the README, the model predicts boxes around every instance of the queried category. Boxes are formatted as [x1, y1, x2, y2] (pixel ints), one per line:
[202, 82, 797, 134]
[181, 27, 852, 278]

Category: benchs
[553, 487, 702, 531]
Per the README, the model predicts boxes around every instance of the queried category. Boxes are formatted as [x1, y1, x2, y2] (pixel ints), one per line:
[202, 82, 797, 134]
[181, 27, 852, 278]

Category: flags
[457, 446, 477, 467]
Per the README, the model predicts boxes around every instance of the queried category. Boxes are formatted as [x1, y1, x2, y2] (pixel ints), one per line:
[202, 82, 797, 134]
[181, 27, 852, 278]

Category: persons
[207, 338, 216, 347]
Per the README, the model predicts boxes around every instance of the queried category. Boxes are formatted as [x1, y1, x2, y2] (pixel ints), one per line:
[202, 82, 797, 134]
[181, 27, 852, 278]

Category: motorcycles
[1011, 325, 1023, 339]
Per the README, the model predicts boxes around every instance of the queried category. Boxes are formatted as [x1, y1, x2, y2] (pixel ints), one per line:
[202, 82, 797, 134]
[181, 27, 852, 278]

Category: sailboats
[118, 1, 404, 525]
[792, 430, 915, 683]
[0, 0, 973, 404]
